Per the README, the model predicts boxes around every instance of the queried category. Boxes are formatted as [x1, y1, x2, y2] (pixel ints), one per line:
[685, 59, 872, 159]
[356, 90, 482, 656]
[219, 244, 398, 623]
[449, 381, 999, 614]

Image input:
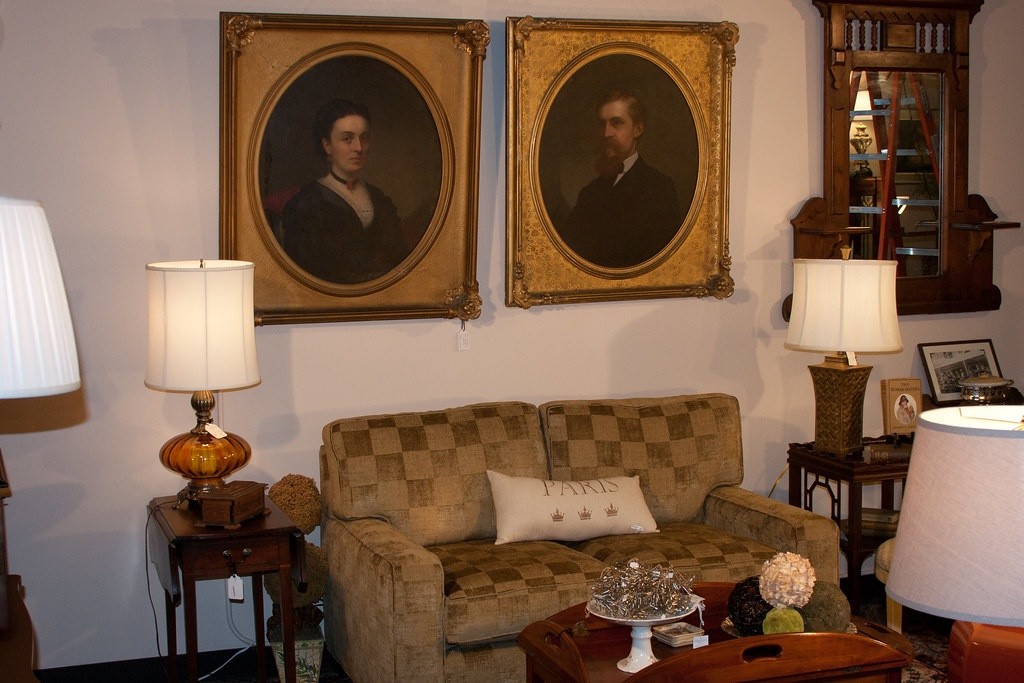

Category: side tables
[146, 494, 307, 683]
[786, 435, 913, 616]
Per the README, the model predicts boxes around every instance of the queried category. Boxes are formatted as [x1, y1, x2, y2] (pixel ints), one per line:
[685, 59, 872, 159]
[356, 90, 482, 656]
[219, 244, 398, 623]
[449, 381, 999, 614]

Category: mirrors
[782, 1, 1020, 324]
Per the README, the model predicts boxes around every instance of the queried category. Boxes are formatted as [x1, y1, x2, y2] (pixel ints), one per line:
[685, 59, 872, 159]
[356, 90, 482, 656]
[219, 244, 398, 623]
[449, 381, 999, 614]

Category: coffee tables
[515, 582, 915, 683]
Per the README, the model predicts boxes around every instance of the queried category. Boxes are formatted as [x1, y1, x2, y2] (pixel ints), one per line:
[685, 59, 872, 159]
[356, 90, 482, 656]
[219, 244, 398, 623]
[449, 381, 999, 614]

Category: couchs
[318, 393, 840, 683]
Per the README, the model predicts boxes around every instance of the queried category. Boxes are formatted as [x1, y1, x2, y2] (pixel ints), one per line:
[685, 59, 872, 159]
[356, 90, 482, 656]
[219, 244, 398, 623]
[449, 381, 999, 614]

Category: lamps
[783, 258, 904, 461]
[143, 260, 263, 510]
[884, 405, 1024, 628]
[0, 195, 82, 638]
[849, 90, 874, 177]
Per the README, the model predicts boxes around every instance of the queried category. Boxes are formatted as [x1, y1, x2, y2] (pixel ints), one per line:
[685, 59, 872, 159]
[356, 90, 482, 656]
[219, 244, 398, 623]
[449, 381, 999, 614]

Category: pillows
[486, 469, 660, 546]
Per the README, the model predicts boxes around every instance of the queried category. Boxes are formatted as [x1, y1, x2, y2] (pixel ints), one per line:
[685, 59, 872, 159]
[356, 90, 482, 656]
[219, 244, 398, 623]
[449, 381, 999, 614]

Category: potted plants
[264, 473, 330, 683]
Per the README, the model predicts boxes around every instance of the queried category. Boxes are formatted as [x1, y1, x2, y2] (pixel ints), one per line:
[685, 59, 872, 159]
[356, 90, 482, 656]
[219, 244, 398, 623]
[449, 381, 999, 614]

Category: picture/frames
[504, 15, 740, 310]
[219, 11, 491, 328]
[917, 338, 1003, 407]
